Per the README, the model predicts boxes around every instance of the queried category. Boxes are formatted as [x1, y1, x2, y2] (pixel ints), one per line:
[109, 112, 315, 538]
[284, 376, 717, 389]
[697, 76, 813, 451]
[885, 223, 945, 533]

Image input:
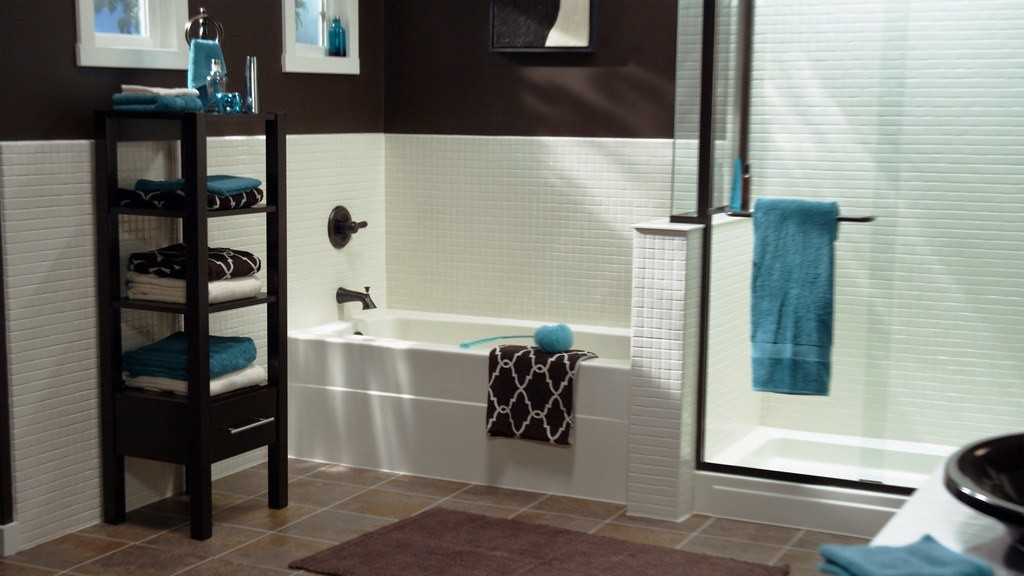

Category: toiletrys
[328, 16, 346, 56]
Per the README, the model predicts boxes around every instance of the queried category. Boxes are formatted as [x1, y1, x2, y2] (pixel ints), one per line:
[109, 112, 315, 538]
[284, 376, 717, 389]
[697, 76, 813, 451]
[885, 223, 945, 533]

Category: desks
[869, 474, 1024, 575]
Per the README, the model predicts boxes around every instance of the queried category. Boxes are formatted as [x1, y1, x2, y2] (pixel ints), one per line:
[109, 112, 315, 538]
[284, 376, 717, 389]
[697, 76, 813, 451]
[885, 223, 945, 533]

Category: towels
[118, 188, 263, 211]
[135, 175, 261, 197]
[486, 344, 599, 447]
[121, 84, 200, 97]
[187, 38, 226, 108]
[128, 271, 261, 303]
[122, 331, 257, 381]
[112, 93, 202, 113]
[123, 365, 267, 397]
[750, 198, 839, 396]
[128, 243, 261, 281]
[819, 534, 994, 576]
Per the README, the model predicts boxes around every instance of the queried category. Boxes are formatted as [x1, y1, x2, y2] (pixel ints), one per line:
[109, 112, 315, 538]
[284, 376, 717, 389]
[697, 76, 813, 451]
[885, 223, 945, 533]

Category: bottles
[206, 58, 226, 113]
[328, 15, 346, 57]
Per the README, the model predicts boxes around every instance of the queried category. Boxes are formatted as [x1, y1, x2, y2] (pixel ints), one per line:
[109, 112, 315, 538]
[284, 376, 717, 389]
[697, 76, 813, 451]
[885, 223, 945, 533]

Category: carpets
[285, 508, 789, 576]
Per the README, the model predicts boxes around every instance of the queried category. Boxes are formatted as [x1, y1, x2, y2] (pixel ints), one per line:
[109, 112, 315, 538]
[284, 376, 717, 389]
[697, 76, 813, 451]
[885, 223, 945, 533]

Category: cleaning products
[206, 59, 225, 112]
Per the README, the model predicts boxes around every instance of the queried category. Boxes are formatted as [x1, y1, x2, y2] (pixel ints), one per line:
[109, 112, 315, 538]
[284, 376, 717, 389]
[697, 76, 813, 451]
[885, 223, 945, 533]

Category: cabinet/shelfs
[92, 109, 288, 540]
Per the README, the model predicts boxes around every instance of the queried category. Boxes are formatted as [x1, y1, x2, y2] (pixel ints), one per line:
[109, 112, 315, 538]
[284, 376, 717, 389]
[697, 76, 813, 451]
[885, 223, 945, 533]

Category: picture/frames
[489, 0, 599, 53]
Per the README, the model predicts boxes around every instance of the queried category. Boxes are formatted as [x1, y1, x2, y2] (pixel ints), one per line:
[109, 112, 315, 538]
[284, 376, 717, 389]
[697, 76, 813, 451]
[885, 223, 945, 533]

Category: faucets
[336, 287, 377, 310]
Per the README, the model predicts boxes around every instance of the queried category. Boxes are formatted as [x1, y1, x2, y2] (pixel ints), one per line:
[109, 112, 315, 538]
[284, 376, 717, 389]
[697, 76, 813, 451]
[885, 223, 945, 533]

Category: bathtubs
[289, 309, 631, 504]
